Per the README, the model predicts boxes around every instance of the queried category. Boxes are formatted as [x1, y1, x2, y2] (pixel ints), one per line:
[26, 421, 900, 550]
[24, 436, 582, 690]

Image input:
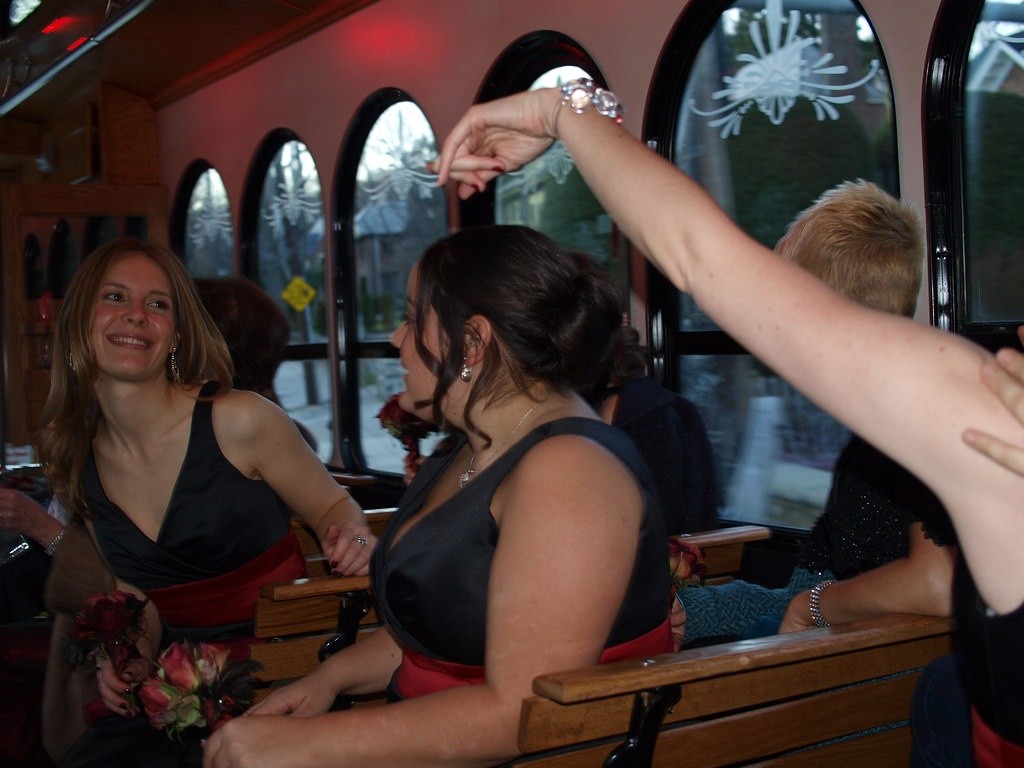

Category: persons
[675, 178, 954, 647]
[33, 234, 382, 768]
[427, 80, 1023, 767]
[200, 220, 682, 767]
[0, 265, 317, 560]
[403, 253, 727, 652]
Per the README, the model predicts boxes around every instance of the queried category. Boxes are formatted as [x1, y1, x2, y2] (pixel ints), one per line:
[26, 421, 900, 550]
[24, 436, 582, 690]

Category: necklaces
[457, 397, 543, 488]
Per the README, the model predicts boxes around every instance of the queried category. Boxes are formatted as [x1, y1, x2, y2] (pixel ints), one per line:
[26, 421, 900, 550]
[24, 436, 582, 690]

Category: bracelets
[45, 528, 65, 555]
[809, 580, 837, 627]
[552, 77, 625, 127]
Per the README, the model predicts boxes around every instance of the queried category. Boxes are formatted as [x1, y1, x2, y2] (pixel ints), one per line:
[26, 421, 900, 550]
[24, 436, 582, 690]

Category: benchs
[250, 477, 949, 768]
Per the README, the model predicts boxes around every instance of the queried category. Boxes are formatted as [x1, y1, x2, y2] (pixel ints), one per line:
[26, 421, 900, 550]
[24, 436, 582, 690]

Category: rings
[353, 535, 366, 546]
